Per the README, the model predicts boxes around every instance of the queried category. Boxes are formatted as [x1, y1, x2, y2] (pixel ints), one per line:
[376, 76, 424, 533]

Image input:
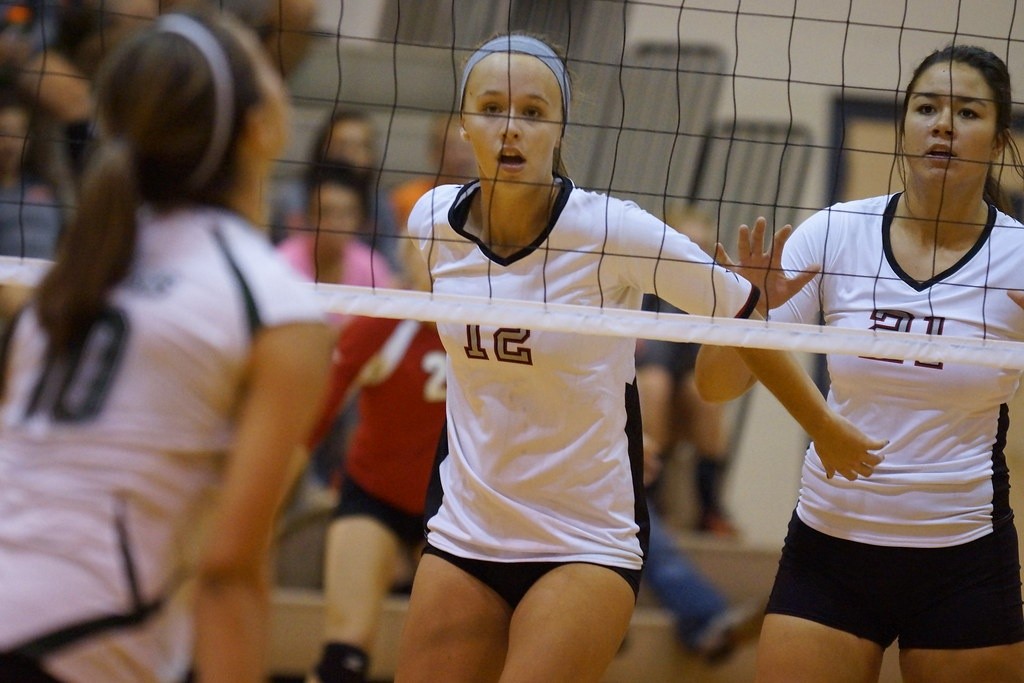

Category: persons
[694, 45, 1024, 683]
[280, 182, 390, 289]
[636, 216, 773, 662]
[0, 13, 339, 683]
[0, 0, 320, 330]
[290, 275, 450, 683]
[392, 32, 890, 683]
[274, 109, 397, 262]
[382, 112, 479, 230]
